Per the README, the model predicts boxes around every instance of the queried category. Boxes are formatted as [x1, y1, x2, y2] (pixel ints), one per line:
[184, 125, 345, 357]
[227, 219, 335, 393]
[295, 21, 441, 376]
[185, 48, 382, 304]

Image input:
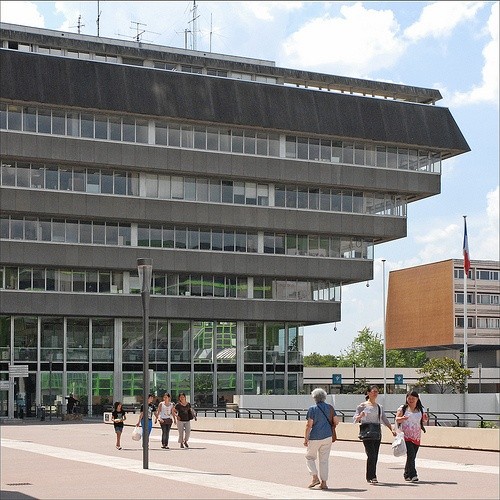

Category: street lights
[136, 257, 153, 468]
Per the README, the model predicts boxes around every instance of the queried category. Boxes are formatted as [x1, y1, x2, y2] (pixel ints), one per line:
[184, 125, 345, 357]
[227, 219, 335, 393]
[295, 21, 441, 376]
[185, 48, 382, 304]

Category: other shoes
[161, 446, 169, 449]
[184, 442, 188, 447]
[115, 446, 122, 450]
[308, 481, 320, 488]
[367, 478, 379, 483]
[180, 445, 184, 448]
[321, 484, 328, 489]
[405, 476, 418, 481]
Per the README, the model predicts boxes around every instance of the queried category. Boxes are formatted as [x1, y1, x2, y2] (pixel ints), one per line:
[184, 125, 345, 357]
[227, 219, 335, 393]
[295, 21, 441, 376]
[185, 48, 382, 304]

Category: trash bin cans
[38, 407, 45, 421]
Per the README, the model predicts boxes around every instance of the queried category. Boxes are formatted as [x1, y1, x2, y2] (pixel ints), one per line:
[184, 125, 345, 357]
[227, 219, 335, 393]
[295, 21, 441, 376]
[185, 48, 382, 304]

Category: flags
[463, 218, 470, 280]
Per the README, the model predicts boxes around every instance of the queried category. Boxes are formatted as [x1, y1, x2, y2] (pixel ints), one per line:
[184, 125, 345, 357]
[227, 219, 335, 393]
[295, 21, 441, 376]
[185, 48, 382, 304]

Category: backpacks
[394, 404, 426, 433]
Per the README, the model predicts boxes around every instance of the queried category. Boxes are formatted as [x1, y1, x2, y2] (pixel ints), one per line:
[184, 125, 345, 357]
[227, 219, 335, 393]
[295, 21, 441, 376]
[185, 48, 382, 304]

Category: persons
[136, 395, 157, 448]
[353, 386, 397, 484]
[58, 393, 80, 421]
[305, 387, 338, 491]
[155, 393, 176, 449]
[173, 393, 193, 448]
[111, 402, 126, 450]
[395, 391, 426, 483]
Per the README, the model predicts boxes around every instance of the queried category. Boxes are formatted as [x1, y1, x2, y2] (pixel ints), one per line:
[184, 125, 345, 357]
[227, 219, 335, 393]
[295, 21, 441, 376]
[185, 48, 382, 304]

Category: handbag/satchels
[358, 423, 381, 441]
[188, 403, 193, 420]
[391, 432, 407, 457]
[331, 422, 337, 442]
[160, 419, 172, 425]
